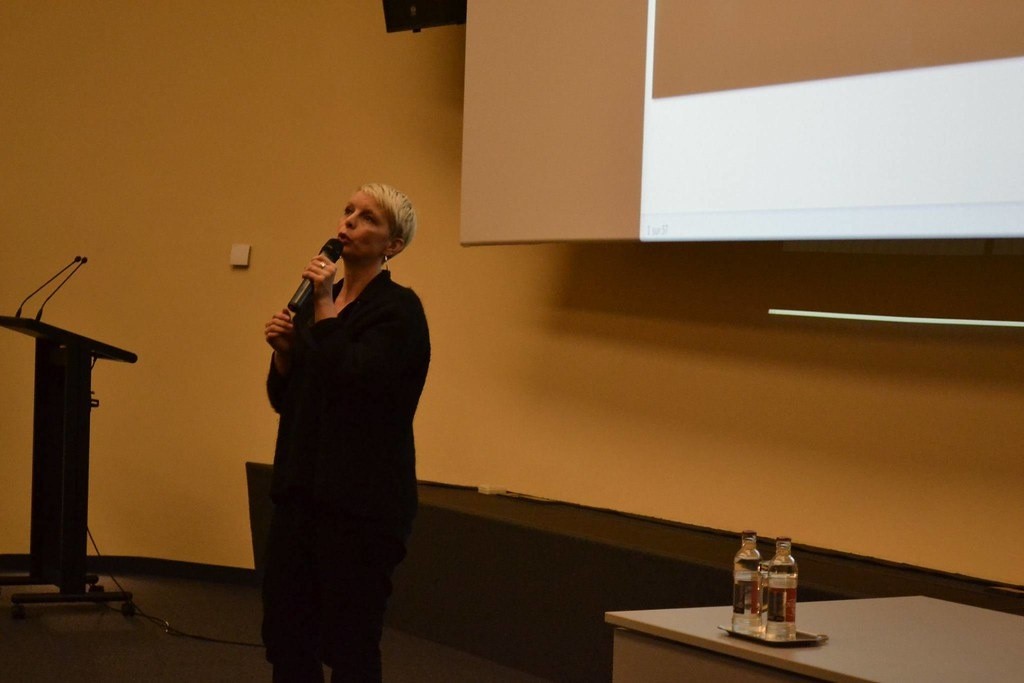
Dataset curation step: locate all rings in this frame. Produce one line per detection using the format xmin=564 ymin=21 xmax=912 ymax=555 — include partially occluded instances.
xmin=320 ymin=261 xmax=326 ymax=269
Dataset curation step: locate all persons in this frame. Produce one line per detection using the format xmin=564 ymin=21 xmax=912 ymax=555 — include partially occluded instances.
xmin=258 ymin=185 xmax=432 ymax=683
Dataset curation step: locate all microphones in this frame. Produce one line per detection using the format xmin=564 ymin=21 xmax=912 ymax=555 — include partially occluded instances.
xmin=288 ymin=238 xmax=343 ymax=314
xmin=36 ymin=257 xmax=88 ymax=321
xmin=15 ymin=256 xmax=81 ymax=318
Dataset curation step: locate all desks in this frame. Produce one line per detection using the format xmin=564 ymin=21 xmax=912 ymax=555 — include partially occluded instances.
xmin=604 ymin=594 xmax=1024 ymax=682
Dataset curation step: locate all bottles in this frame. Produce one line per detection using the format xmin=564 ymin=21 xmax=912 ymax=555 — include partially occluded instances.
xmin=766 ymin=535 xmax=798 ymax=640
xmin=732 ymin=529 xmax=765 ymax=637
xmin=754 ymin=557 xmax=774 ymax=636
